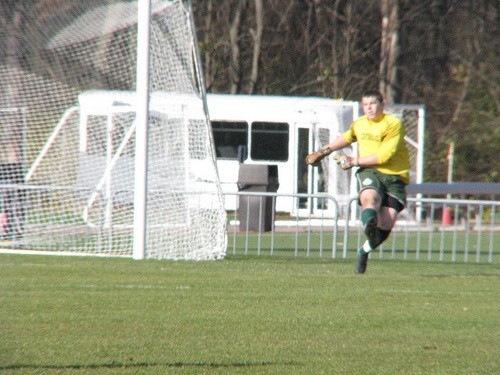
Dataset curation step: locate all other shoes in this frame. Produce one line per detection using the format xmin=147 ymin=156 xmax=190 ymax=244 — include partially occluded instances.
xmin=362 ymin=216 xmax=377 ymax=241
xmin=355 ymin=247 xmax=367 ymax=274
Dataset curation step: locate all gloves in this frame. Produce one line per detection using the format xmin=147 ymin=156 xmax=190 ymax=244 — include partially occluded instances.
xmin=333 ymin=154 xmax=359 ymax=170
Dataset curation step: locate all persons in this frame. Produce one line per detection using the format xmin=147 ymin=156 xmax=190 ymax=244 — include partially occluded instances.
xmin=306 ymin=90 xmax=410 ymax=273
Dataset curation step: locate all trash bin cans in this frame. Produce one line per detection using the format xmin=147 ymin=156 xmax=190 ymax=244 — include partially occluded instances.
xmin=0 ymin=161 xmax=27 ymax=241
xmin=235 ymin=163 xmax=280 ymax=233
